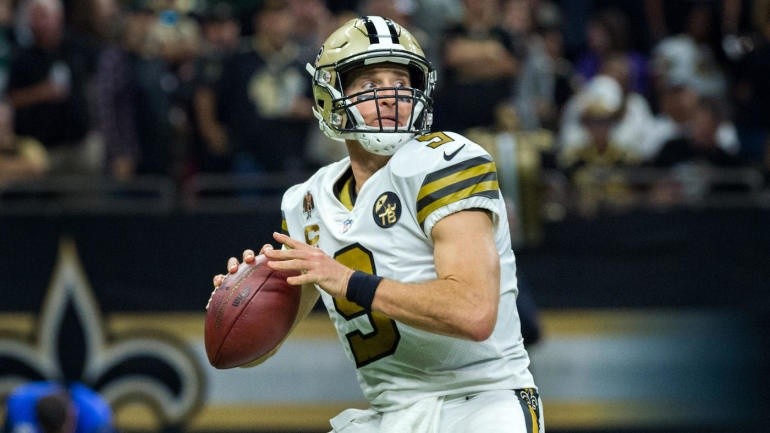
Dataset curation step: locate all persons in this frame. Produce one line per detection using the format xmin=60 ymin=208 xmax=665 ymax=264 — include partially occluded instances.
xmin=213 ymin=15 xmax=545 ymax=433
xmin=0 ymin=0 xmax=770 ymax=213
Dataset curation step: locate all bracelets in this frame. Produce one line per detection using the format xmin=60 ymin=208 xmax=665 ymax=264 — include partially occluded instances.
xmin=345 ymin=269 xmax=384 ymax=312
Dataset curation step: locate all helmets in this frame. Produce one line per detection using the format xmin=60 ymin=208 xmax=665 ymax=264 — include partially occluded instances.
xmin=576 ymin=75 xmax=622 ymax=123
xmin=652 ymin=36 xmax=699 ymax=90
xmin=312 ymin=15 xmax=437 ymax=155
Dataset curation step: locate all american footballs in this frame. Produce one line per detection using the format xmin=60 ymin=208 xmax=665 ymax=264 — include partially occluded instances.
xmin=204 ymin=249 xmax=301 ymax=370
